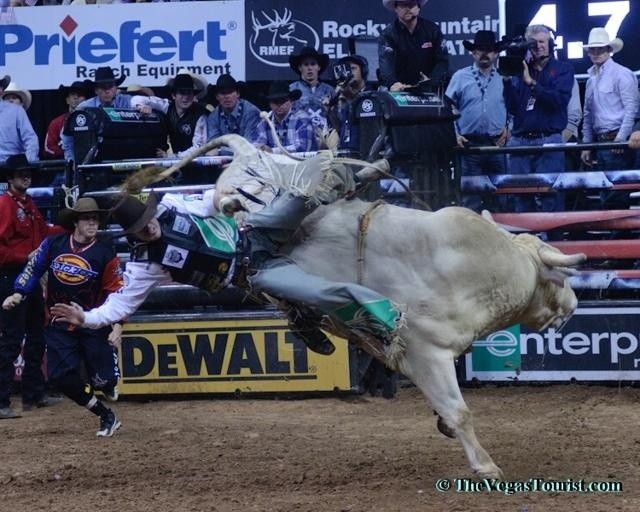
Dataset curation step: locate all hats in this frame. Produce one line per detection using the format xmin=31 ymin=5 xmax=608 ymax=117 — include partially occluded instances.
xmin=108 ymin=191 xmax=158 ymax=238
xmin=463 ymin=30 xmax=511 ymax=53
xmin=58 ymin=67 xmax=126 ymax=97
xmin=260 ymin=81 xmax=301 ymax=102
xmin=207 ymin=73 xmax=247 ymax=94
xmin=168 ymin=73 xmax=204 ymax=96
xmin=0 ymin=75 xmax=32 ymax=111
xmin=1 ymin=154 xmax=41 ymax=183
xmin=59 ymin=198 xmax=110 ymax=230
xmin=290 ymin=48 xmax=329 ymax=74
xmin=383 ymin=0 xmax=427 ymax=12
xmin=582 ymin=28 xmax=624 ymax=54
xmin=343 ymin=54 xmax=369 ymax=80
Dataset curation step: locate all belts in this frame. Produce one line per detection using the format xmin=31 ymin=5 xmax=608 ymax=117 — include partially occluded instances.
xmin=522 ymin=130 xmax=556 ymax=140
xmin=597 ymin=129 xmax=619 ymax=141
xmin=212 ymin=262 xmax=228 ymax=289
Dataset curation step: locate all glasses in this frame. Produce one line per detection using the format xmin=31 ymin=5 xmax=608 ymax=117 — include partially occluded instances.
xmin=396 ymin=1 xmax=417 ymax=8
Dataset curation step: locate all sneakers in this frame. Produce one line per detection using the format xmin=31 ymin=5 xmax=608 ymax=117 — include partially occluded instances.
xmin=0 ymin=408 xmax=22 ymax=419
xmin=24 ymin=397 xmax=63 ymax=409
xmin=96 ymin=409 xmax=122 ymax=438
xmin=104 ymin=385 xmax=119 ymax=402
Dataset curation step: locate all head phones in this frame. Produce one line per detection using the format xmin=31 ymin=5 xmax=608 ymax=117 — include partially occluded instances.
xmin=352 ymin=53 xmax=369 ymax=81
xmin=542 ymin=24 xmax=557 ymax=57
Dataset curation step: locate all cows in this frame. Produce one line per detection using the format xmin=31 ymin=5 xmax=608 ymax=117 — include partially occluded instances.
xmin=111 ymin=132 xmax=589 ymax=482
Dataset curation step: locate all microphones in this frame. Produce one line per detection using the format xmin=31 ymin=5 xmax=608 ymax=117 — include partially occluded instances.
xmin=540 ymin=54 xmax=550 ymax=61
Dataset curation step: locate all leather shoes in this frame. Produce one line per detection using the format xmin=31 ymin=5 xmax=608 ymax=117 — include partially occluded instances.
xmin=355 ymin=159 xmax=390 ymax=186
xmin=289 ymin=320 xmax=335 ymax=354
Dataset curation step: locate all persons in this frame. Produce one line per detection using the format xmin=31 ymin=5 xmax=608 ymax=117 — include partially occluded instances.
xmin=0 ymin=1 xmax=639 ymax=239
xmin=0 ymin=156 xmax=65 ymax=419
xmin=49 ymin=158 xmax=391 ymax=355
xmin=3 ymin=196 xmax=125 ymax=437
